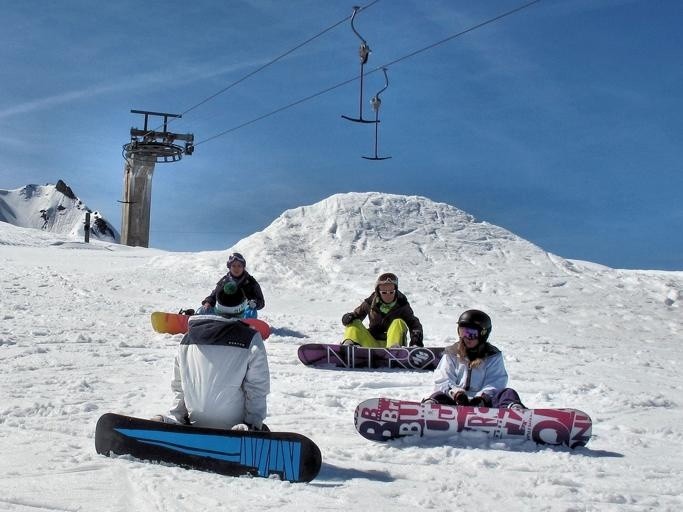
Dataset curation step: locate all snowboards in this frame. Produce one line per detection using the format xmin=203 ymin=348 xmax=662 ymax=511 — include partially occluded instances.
xmin=298 ymin=344 xmax=446 ymax=373
xmin=151 ymin=310 xmax=272 ymax=340
xmin=94 ymin=413 xmax=321 ymax=482
xmin=353 ymin=399 xmax=592 ymax=450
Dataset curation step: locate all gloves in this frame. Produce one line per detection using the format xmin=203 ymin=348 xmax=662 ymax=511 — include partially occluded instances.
xmin=342 ymin=313 xmax=355 ymax=325
xmin=249 ymin=299 xmax=257 ymax=309
xmin=454 ymin=392 xmax=469 ymax=406
xmin=409 ymin=336 xmax=423 ymax=347
xmin=469 ymin=396 xmax=488 ymax=408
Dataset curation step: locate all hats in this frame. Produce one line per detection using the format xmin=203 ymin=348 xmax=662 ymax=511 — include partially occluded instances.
xmin=213 ymin=282 xmax=248 ymax=315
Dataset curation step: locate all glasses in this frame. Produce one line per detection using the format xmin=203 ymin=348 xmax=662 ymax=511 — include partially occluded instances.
xmin=458 ymin=326 xmax=488 ymax=339
xmin=229 ymin=253 xmax=245 ymax=263
xmin=379 ymin=291 xmax=394 ymax=295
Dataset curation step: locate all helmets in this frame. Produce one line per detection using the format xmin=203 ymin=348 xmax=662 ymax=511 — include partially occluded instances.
xmin=227 ymin=253 xmax=246 ymax=269
xmin=375 ymin=273 xmax=398 ymax=290
xmin=457 ymin=310 xmax=491 ymax=345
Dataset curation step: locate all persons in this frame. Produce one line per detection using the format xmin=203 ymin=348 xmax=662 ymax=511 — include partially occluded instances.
xmin=198 ymin=250 xmax=266 ymax=319
xmin=419 ymin=307 xmax=524 ymax=409
xmin=151 ymin=281 xmax=271 ymax=435
xmin=339 ymin=273 xmax=424 ymax=349
